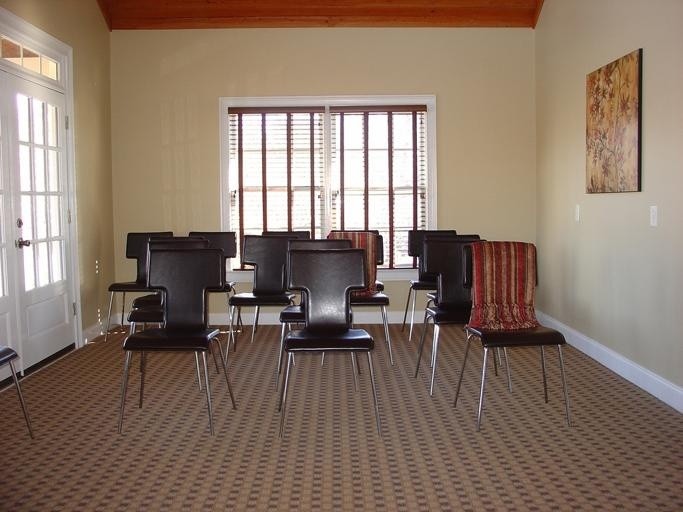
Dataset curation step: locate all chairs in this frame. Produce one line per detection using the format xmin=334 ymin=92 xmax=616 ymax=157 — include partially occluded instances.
xmin=450 ymin=240 xmax=573 ymax=433
xmin=0 ymin=345 xmax=35 ymax=439
xmin=102 ymin=230 xmax=574 ymax=391
xmin=276 ymin=246 xmax=387 ymax=437
xmin=115 ymin=246 xmax=237 ymax=440
